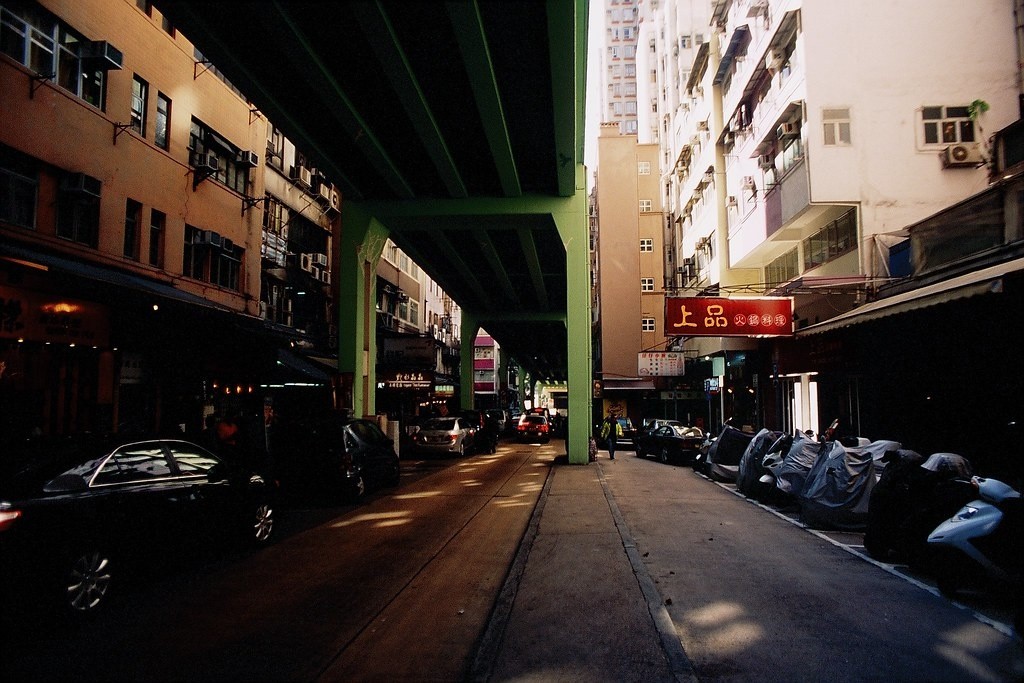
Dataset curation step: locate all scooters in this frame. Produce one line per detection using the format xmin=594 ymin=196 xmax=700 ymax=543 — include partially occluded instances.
xmin=692 ymin=417 xmax=979 ymax=574
xmin=927 ymin=474 xmax=1024 ymax=594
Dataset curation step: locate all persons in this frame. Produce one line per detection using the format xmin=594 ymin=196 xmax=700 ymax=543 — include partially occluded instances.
xmin=600 ymin=411 xmax=623 ymax=460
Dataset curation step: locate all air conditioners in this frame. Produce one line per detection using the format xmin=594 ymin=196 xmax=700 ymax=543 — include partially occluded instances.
xmin=329 ymin=190 xmax=338 ymax=207
xmin=294 ymin=166 xmax=311 ymax=188
xmin=316 ymin=183 xmax=330 ymax=201
xmin=675 ymin=85 xmax=713 ymax=217
xmin=194 ymin=230 xmax=220 ymax=248
xmin=64 ymin=172 xmax=103 ymax=199
xmin=198 ymin=154 xmax=218 ymax=172
xmin=740 ymin=176 xmax=754 ymax=190
xmin=724 ymin=134 xmax=733 ymax=144
xmin=945 ymin=144 xmax=983 ymax=166
xmin=677 ymin=266 xmax=684 ymax=273
xmin=82 ymin=40 xmax=123 ymax=70
xmin=220 ymin=237 xmax=233 ymax=252
xmin=730 ymin=118 xmax=739 ymax=132
xmin=777 ymin=122 xmax=798 ymax=140
xmin=296 ymin=253 xmax=311 ymax=272
xmin=241 ymin=150 xmax=258 ymax=168
xmin=757 ymin=155 xmax=775 ymax=168
xmin=683 ymin=258 xmax=691 ymax=265
xmin=314 ymin=253 xmax=327 ymax=266
xmin=766 ymin=50 xmax=784 ymax=69
xmin=726 ymin=196 xmax=735 ymax=207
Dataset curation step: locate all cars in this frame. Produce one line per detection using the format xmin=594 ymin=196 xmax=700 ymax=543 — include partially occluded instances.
xmin=0 ymin=431 xmax=280 ymax=628
xmin=597 ymin=416 xmax=710 ymax=465
xmin=472 ymin=407 xmax=554 ymax=455
xmin=267 ymin=419 xmax=402 ymax=505
xmin=411 ymin=415 xmax=478 ymax=458
